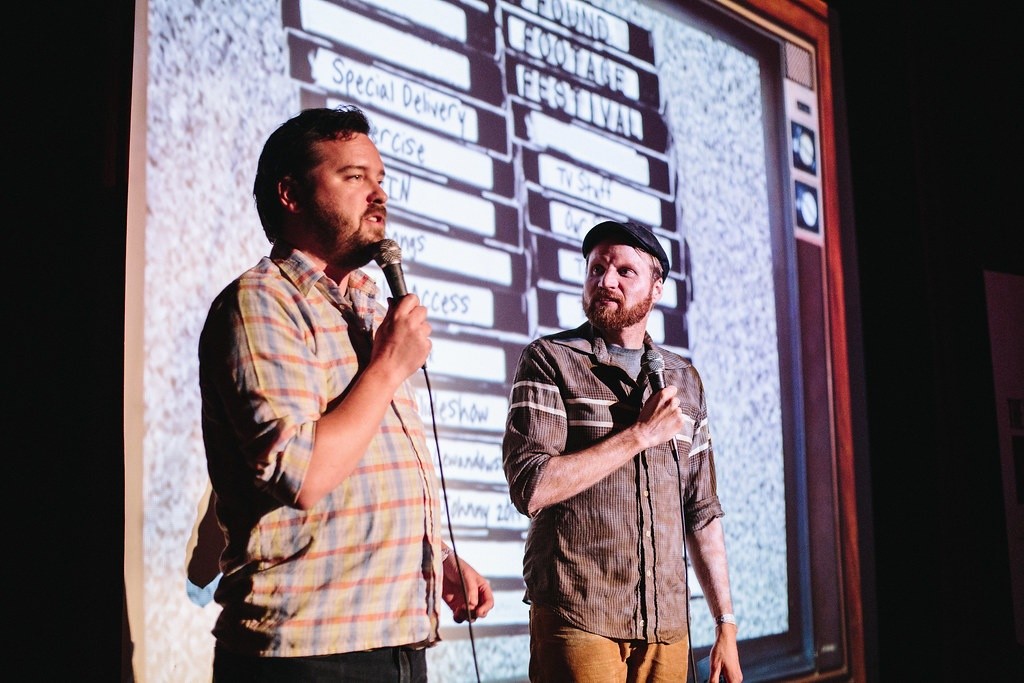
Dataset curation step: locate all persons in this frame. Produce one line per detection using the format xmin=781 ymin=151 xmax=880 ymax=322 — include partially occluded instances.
xmin=503 ymin=222 xmax=743 ymax=683
xmin=198 ymin=106 xmax=494 ymax=683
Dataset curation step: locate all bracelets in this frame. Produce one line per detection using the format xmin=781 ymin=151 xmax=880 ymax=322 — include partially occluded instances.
xmin=714 ymin=615 xmax=737 ymax=627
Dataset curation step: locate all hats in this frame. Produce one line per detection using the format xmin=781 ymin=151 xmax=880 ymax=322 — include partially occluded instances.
xmin=582 ymin=221 xmax=670 ymax=284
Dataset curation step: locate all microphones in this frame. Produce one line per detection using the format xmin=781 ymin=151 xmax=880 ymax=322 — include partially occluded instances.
xmin=642 ymin=350 xmax=679 ymax=462
xmin=375 ymin=239 xmax=428 ymax=370
